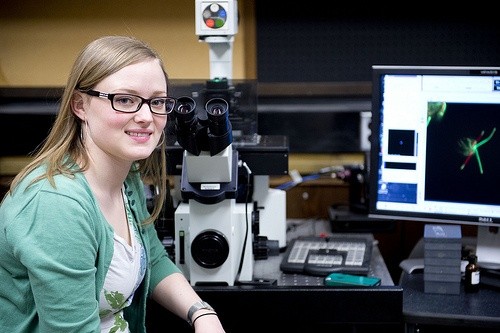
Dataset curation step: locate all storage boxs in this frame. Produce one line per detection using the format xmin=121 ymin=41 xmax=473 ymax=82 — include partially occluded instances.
xmin=424 ymin=224 xmax=461 ymax=295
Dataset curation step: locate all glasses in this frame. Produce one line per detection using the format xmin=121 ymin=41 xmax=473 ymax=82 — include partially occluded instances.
xmin=81 ymin=87 xmax=177 ymax=115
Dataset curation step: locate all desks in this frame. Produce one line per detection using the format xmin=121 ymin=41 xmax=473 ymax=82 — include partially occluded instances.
xmin=145 ymin=234 xmax=404 ymax=333
xmin=399 ymin=239 xmax=500 ymax=321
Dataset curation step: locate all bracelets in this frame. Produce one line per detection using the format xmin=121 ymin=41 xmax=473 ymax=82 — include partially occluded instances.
xmin=187 ymin=301 xmax=214 ymax=326
xmin=192 ymin=312 xmax=217 ymax=327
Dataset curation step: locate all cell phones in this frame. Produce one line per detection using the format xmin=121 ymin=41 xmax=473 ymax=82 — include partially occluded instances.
xmin=324 ymin=272 xmax=382 ymax=289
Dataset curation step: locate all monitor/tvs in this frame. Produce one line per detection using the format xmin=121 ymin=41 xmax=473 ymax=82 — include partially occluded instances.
xmin=367 ymin=66 xmax=500 ymax=276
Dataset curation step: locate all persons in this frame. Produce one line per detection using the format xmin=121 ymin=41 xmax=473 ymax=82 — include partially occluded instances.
xmin=0 ymin=35 xmax=225 ymax=333
